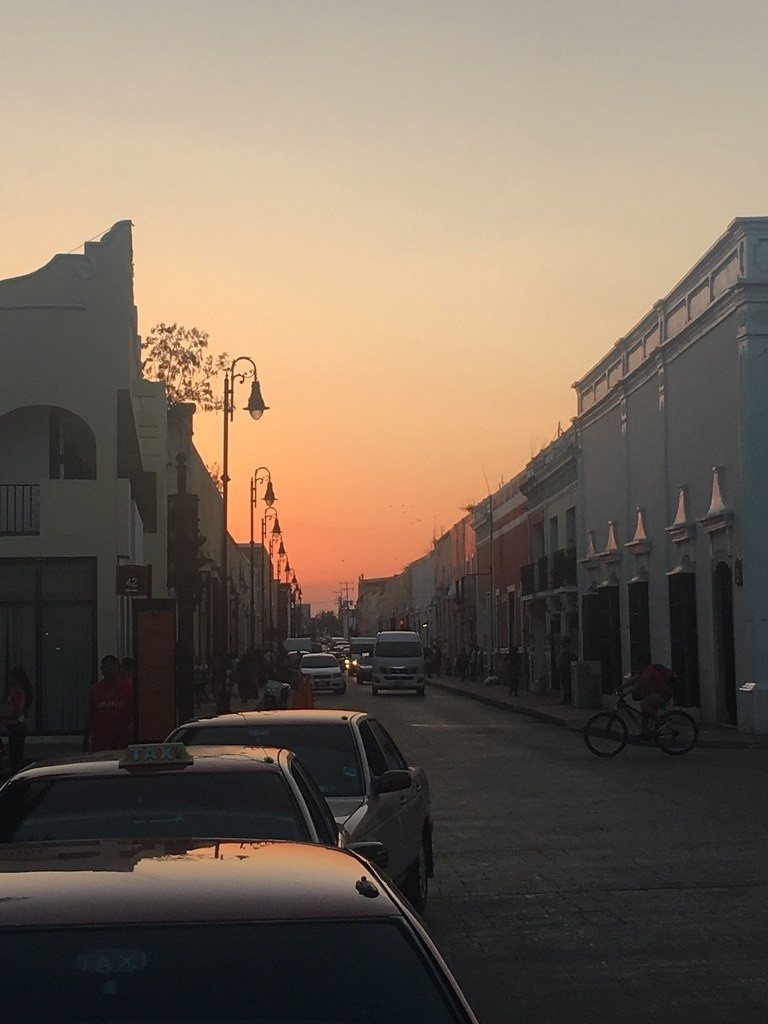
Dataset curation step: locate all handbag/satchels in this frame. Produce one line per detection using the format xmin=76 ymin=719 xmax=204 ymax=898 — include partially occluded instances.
xmin=81 ymin=735 xmax=101 ymax=753
xmin=654 ymin=663 xmax=676 ymax=686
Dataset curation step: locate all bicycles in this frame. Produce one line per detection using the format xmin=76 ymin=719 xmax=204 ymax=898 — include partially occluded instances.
xmin=582 ymin=676 xmax=702 ymax=757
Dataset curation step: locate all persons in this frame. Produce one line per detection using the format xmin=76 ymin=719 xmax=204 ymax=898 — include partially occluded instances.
xmin=190 ymin=654 xmax=261 ymax=714
xmin=83 ymin=650 xmax=134 ymax=751
xmin=558 ymin=636 xmax=579 ymax=705
xmin=6 ymin=665 xmax=34 ymax=775
xmin=504 ymin=644 xmax=523 ymax=697
xmin=427 ymin=645 xmax=443 ymax=679
xmin=456 ymin=647 xmax=467 ymax=682
xmin=615 ymin=656 xmax=677 ymax=738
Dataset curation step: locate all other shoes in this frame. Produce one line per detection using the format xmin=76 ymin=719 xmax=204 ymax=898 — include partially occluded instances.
xmin=642 ymin=736 xmax=656 ymax=744
xmin=508 ymin=688 xmax=513 ymax=696
xmin=515 ymin=693 xmax=519 ymax=696
xmin=427 ymin=676 xmax=432 ymax=678
xmin=437 ymin=675 xmax=440 ymax=677
xmin=654 ymin=720 xmax=668 ymax=732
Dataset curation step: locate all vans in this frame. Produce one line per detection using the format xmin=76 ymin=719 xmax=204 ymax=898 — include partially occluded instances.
xmin=346 ymin=637 xmax=380 ymax=676
xmin=372 ymin=632 xmax=433 ymax=694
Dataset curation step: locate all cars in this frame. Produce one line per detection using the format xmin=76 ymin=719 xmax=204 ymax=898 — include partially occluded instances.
xmin=357 ymin=653 xmax=376 ymax=685
xmin=1 ymin=746 xmax=389 ymax=877
xmin=152 ymin=708 xmax=437 ymax=910
xmin=1 ymin=836 xmax=475 ymax=1023
xmin=311 ymin=635 xmax=349 ymax=670
xmin=299 ymin=653 xmax=348 ymax=693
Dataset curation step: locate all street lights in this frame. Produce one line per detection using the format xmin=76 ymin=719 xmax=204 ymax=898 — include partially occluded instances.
xmin=267 ymin=534 xmax=304 ymax=640
xmin=217 ymin=357 xmax=272 ymax=712
xmin=249 ymin=466 xmax=280 ymax=649
xmin=261 ymin=506 xmax=284 ymax=642
